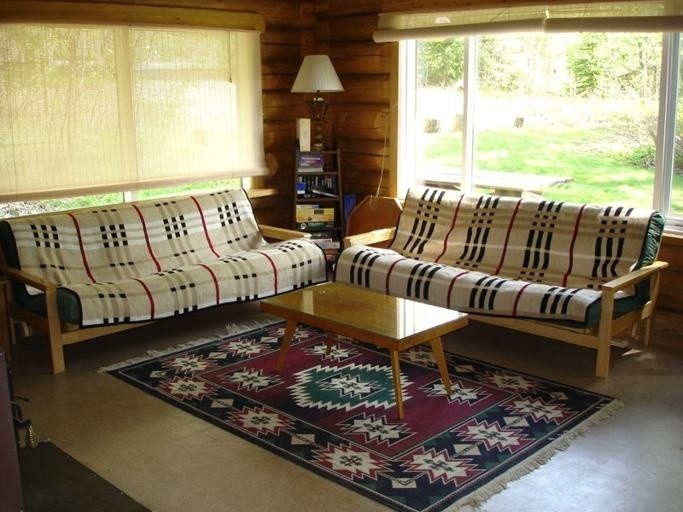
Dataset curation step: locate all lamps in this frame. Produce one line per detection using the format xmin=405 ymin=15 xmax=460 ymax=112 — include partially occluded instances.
xmin=289 ymin=53 xmax=346 ymax=150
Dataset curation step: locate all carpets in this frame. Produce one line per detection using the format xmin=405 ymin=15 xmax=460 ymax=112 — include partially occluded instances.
xmin=20 ymin=435 xmax=151 ymax=511
xmin=94 ymin=312 xmax=624 ymax=511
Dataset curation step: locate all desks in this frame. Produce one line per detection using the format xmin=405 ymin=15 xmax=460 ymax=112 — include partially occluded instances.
xmin=255 ymin=278 xmax=472 ymax=426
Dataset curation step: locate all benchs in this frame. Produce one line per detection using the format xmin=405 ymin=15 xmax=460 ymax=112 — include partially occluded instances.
xmin=332 ymin=180 xmax=671 ymax=381
xmin=1 ymin=186 xmax=329 ymax=377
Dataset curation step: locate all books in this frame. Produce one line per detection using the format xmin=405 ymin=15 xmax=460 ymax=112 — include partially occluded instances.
xmin=297 ymin=155 xmax=338 ymax=198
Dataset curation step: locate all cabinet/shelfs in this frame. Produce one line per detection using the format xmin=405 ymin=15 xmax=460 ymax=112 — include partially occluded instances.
xmin=288 ymin=150 xmax=346 ymax=270
